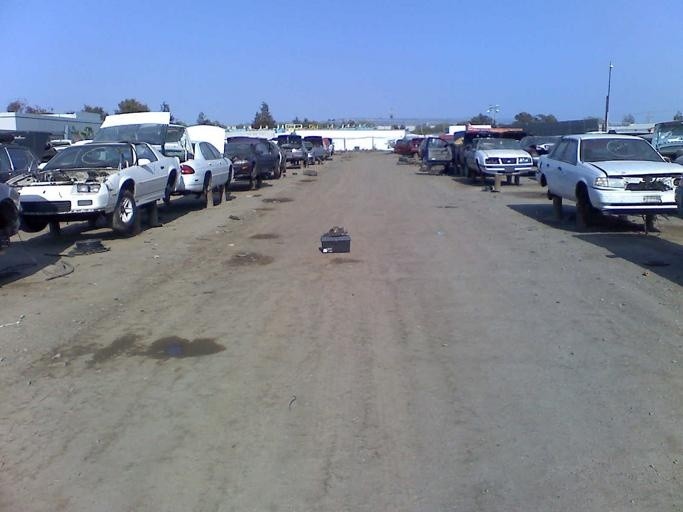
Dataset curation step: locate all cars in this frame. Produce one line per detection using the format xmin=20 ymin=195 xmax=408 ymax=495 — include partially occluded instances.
xmin=133 ymin=124 xmax=333 ymax=202
xmin=0 ymin=181 xmax=21 ymax=246
xmin=0 ymin=130 xmax=50 ymax=182
xmin=533 ymin=129 xmax=683 ymax=218
xmin=48 ymin=136 xmax=89 ymax=151
xmin=392 ymin=120 xmax=683 ymax=178
xmin=6 ymin=111 xmax=181 ymax=234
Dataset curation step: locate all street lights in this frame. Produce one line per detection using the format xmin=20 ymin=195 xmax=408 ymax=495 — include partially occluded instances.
xmin=486 ymin=102 xmax=500 ymax=124
xmin=602 ymin=59 xmax=613 ymax=131
